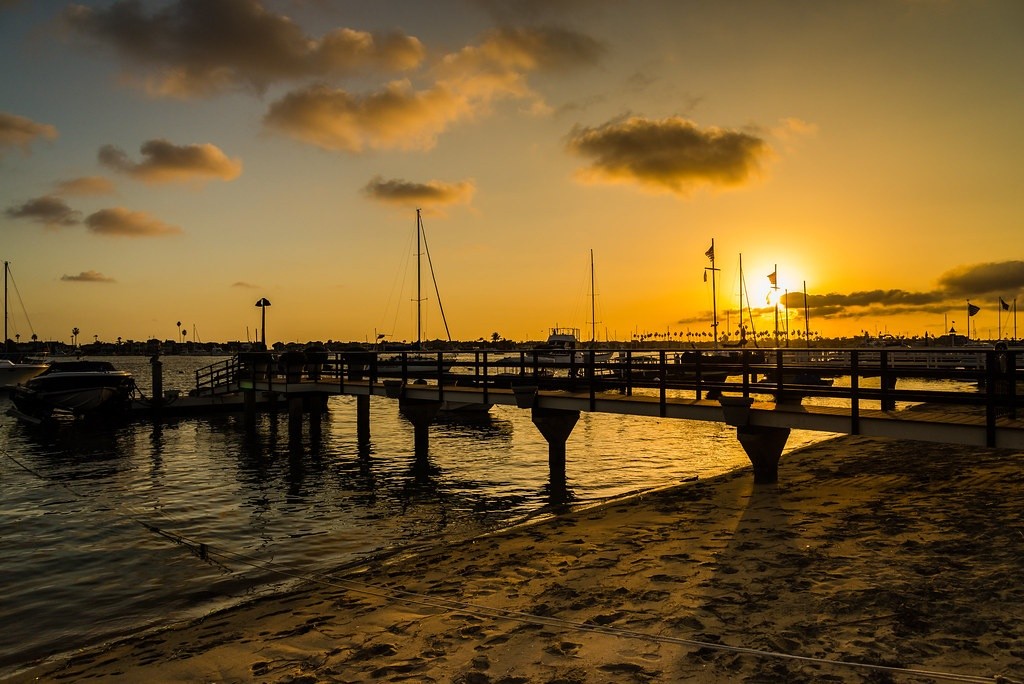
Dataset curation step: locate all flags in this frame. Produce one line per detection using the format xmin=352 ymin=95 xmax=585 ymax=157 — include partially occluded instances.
xmin=969 ymin=304 xmax=980 ymax=316
xmin=706 ymin=247 xmax=714 ymax=261
xmin=767 ymin=272 xmax=776 ymax=284
xmin=1001 ymin=299 xmax=1009 ymax=310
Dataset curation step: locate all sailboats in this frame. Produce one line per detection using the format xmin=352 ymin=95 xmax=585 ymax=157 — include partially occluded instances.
xmin=362 ymin=207 xmax=457 ymax=376
xmin=0 ymin=260 xmax=50 ymax=393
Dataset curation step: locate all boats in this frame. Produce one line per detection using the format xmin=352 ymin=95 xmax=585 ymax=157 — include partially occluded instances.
xmin=398 ymin=374 xmax=502 ymax=412
xmin=497 ymin=238 xmax=1024 ymax=385
xmin=4 ymin=360 xmax=134 ymax=431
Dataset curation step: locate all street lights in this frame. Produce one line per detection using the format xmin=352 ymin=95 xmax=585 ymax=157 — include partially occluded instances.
xmin=255 ymin=297 xmax=272 ymax=344
